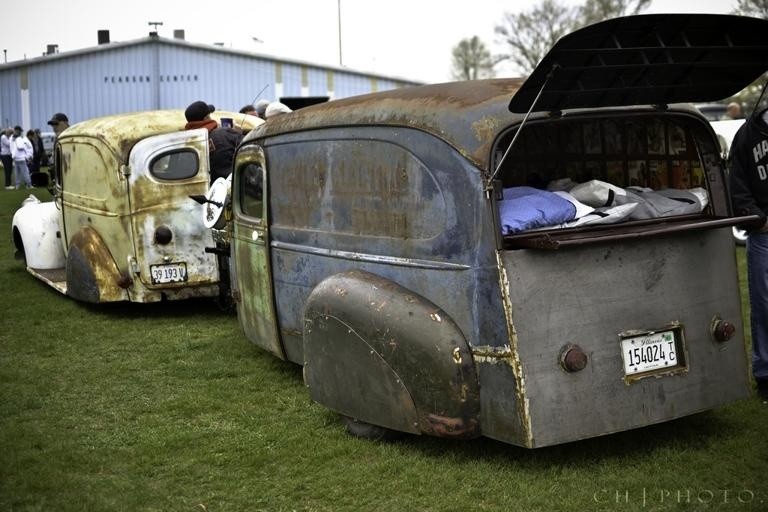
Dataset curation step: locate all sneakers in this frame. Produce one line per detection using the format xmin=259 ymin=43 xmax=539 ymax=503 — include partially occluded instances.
xmin=26 ymin=185 xmax=34 ymax=189
xmin=5 ymin=185 xmax=16 ymax=190
xmin=756 ymin=376 xmax=768 ymax=405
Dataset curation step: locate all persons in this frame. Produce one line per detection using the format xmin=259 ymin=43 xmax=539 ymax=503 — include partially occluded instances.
xmin=47 ymin=112 xmax=70 ymax=186
xmin=720 ymin=102 xmax=740 ymax=120
xmin=0 ymin=125 xmax=48 ymax=190
xmin=171 ymin=101 xmax=243 ymax=187
xmin=726 ymin=107 xmax=767 ymax=408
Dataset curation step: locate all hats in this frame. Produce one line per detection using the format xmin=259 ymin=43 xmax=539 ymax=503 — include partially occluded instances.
xmin=47 ymin=113 xmax=68 ymax=125
xmin=185 ymin=101 xmax=215 ymax=123
xmin=15 ymin=126 xmax=23 ymax=131
